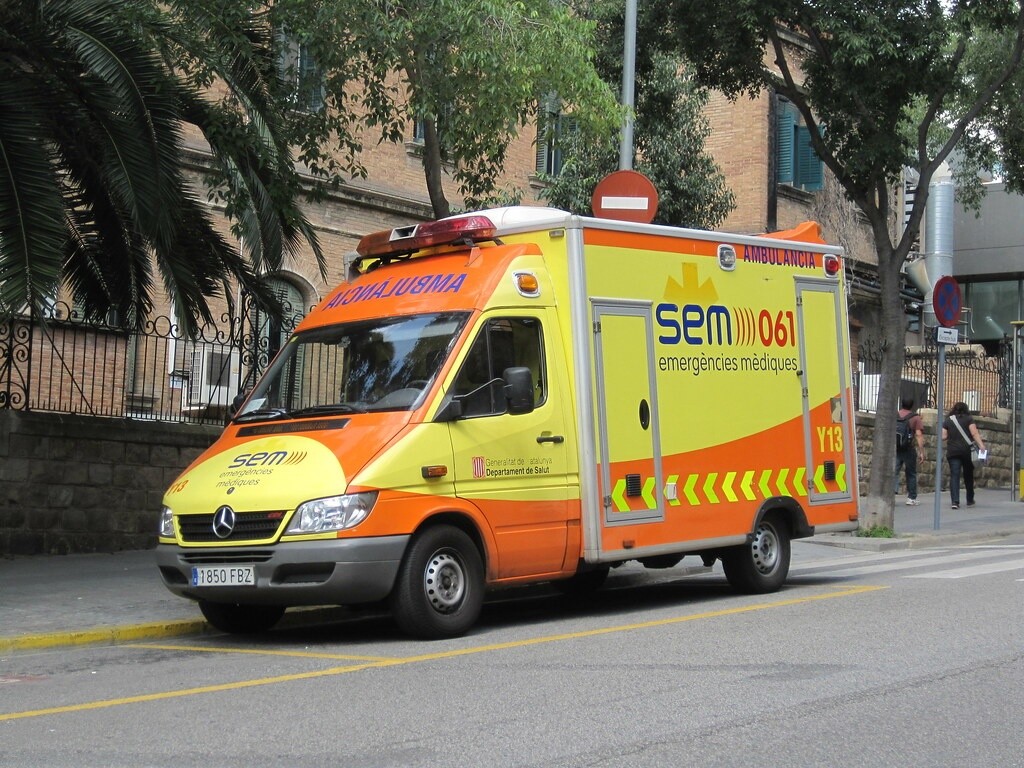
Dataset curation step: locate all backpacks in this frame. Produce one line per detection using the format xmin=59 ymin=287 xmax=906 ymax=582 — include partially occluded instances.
xmin=896 ymin=412 xmax=918 ymax=451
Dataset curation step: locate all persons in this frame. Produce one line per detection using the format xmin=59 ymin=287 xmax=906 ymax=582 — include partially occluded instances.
xmin=895 ymin=397 xmax=924 ymax=505
xmin=941 ymin=402 xmax=985 ymax=509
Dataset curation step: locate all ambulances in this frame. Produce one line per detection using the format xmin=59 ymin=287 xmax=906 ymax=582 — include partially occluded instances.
xmin=153 ymin=206 xmax=861 ymax=639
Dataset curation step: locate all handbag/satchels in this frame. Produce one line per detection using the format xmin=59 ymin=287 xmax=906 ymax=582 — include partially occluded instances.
xmin=971 ymin=441 xmax=982 ymax=468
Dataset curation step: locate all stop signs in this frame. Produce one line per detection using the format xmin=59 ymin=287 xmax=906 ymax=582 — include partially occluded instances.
xmin=591 ymin=172 xmax=658 ymax=225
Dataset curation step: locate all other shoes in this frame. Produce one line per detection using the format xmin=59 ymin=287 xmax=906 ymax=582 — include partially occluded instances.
xmin=968 ymin=503 xmax=975 ymax=507
xmin=952 ymin=502 xmax=959 ymax=509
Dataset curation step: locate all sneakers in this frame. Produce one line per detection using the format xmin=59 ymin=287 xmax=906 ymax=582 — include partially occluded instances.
xmin=906 ymin=497 xmax=920 ymax=506
